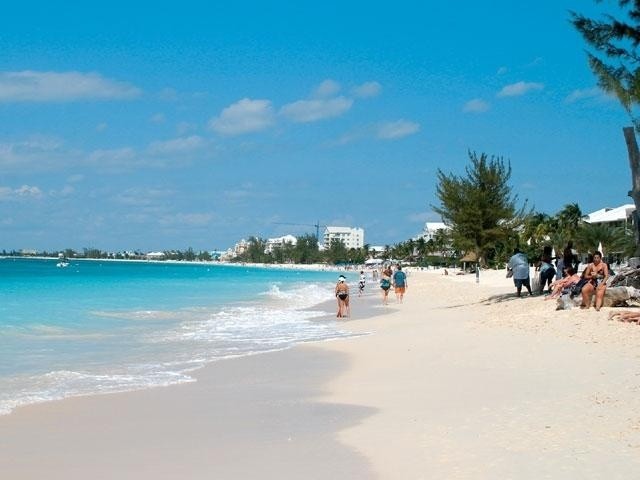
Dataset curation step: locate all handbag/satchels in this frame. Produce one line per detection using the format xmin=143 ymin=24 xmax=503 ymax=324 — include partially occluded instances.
xmin=531 ymin=268 xmax=542 ymax=295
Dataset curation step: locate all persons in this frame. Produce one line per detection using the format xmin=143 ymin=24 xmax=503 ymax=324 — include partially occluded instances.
xmin=335 ymin=277 xmax=348 ymax=318
xmin=506 ymin=237 xmax=611 ymax=310
xmin=336 ymin=275 xmax=351 ymax=318
xmin=358 ymin=260 xmax=409 ymax=303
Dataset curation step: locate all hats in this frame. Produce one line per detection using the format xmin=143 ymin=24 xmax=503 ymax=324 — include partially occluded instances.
xmin=338 ymin=274 xmax=347 ymax=282
